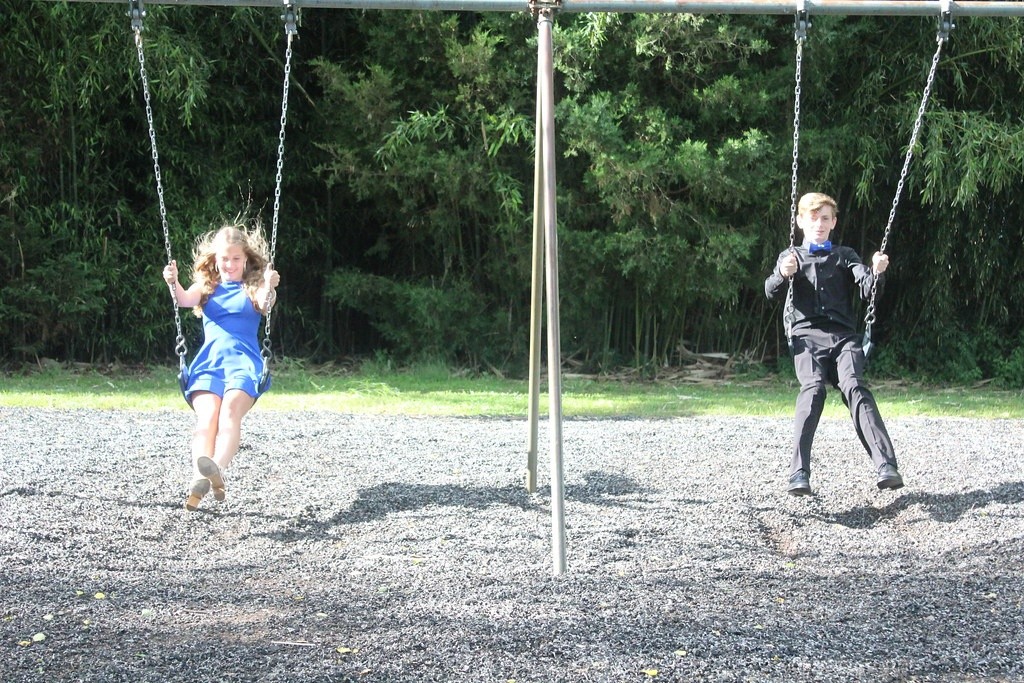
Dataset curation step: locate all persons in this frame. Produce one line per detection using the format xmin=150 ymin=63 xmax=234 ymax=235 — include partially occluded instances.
xmin=764 ymin=191 xmax=904 ymax=495
xmin=162 ymin=221 xmax=281 ymax=511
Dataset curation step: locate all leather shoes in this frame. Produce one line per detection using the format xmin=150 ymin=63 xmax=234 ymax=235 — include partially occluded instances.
xmin=787 ymin=470 xmax=811 ymax=497
xmin=876 ymin=464 xmax=904 ymax=490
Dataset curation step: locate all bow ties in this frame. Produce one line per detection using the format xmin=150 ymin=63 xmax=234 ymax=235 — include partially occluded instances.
xmin=808 ymin=240 xmax=832 ymax=254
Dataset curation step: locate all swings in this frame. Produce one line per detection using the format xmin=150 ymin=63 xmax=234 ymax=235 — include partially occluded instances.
xmin=785 ymin=17 xmax=954 ymax=382
xmin=121 ymin=1 xmax=302 ymax=417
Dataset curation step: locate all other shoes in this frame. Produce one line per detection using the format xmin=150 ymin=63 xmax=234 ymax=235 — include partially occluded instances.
xmin=186 ymin=478 xmax=210 ymax=510
xmin=197 ymin=456 xmax=225 ymax=501
